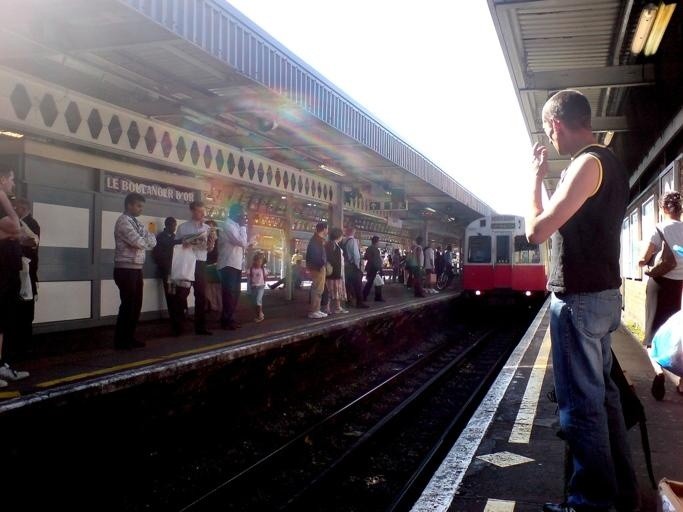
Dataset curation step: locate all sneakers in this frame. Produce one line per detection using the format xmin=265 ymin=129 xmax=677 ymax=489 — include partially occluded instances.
xmin=0 ymin=363 xmax=30 ymax=380
xmin=254 ymin=313 xmax=265 ymax=323
xmin=423 ymin=288 xmax=439 ymax=294
xmin=308 ymin=307 xmax=349 ymax=318
xmin=542 ymin=501 xmax=587 ymax=512
xmin=0 ymin=380 xmax=8 ymax=388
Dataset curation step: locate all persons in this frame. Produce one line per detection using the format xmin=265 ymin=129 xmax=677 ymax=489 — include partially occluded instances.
xmin=1 ymin=163 xmax=40 ymax=388
xmin=523 ymin=85 xmax=645 ymax=511
xmin=111 ymin=193 xmax=269 ymax=348
xmin=268 ymin=221 xmax=455 ymax=318
xmin=637 ymin=189 xmax=683 ymax=402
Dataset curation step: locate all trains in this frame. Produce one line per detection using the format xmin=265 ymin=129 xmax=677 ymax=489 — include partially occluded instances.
xmin=206 ymin=220 xmax=401 ymax=280
xmin=462 ymin=214 xmax=553 ymax=310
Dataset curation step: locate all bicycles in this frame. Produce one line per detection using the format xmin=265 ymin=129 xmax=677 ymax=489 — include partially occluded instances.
xmin=435 ymin=269 xmax=449 ymax=291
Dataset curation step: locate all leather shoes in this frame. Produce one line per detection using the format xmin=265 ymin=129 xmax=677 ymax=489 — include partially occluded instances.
xmin=196 ymin=321 xmax=240 ymax=335
xmin=357 ymin=303 xmax=371 ymax=308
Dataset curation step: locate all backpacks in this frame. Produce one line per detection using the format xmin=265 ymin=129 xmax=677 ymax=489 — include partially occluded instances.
xmin=339 ymin=238 xmax=353 ymax=262
xmin=406 ymin=246 xmax=421 ymax=268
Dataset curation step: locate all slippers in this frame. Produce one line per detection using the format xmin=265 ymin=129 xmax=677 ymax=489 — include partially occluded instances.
xmin=650 ymin=374 xmax=665 ymax=401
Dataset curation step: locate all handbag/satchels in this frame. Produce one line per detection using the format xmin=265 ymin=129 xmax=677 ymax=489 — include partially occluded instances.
xmin=170 ymin=244 xmax=196 ymax=281
xmin=643 ymin=227 xmax=677 ymax=279
xmin=430 ymin=273 xmax=437 ymax=283
xmin=546 ymin=344 xmax=643 ymax=445
xmin=308 ymin=283 xmax=328 ymax=305
